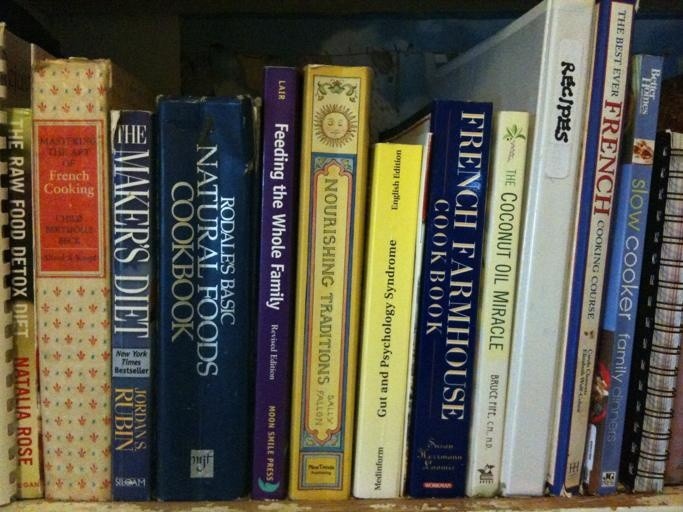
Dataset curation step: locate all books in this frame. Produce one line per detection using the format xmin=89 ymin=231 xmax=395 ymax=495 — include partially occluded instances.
xmin=0 ymin=1 xmax=682 ymax=503
